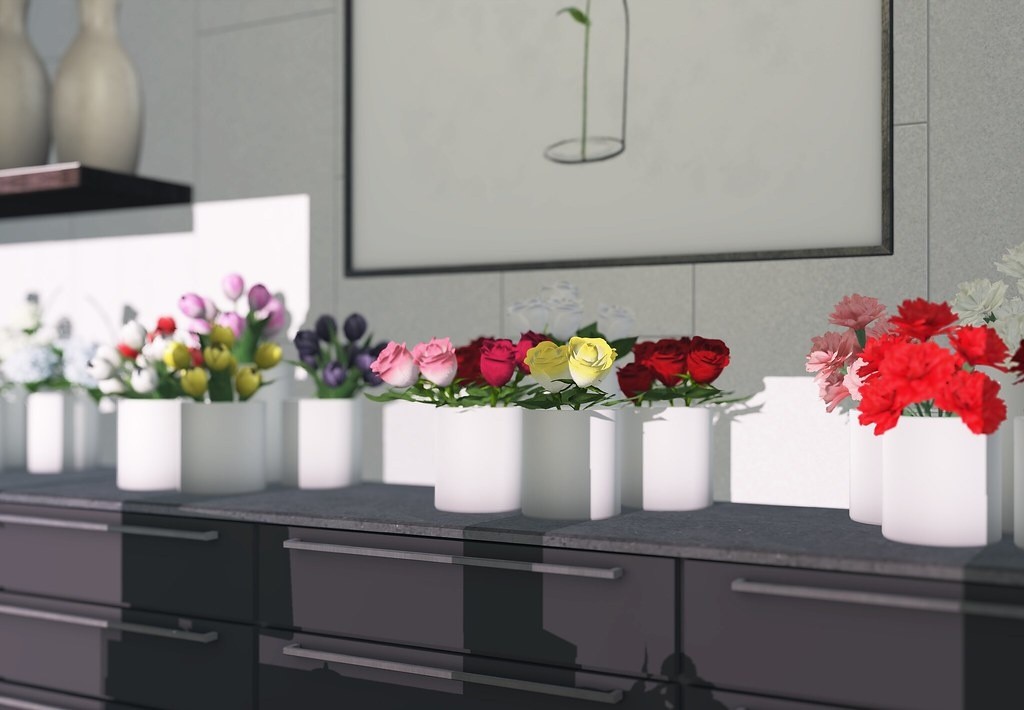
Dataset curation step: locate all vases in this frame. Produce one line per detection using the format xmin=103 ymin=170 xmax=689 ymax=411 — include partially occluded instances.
xmin=0 ymin=377 xmax=1023 ymax=549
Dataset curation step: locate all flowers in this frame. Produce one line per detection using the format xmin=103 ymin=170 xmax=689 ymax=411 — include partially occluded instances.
xmin=2 ymin=243 xmax=1024 ymax=437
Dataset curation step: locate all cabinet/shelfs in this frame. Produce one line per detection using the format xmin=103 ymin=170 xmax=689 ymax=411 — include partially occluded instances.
xmin=0 ymin=502 xmax=1024 ymax=710
